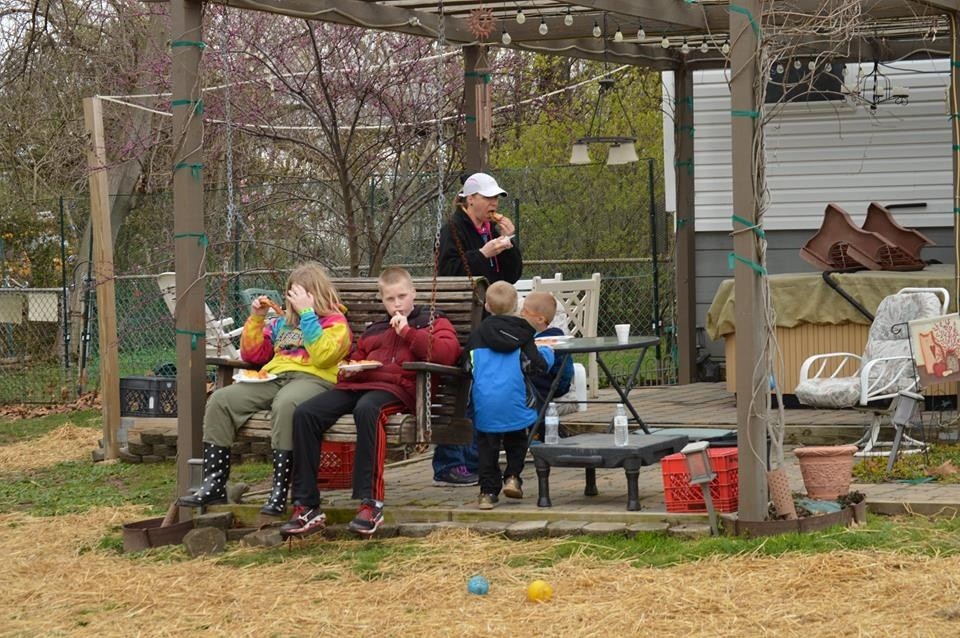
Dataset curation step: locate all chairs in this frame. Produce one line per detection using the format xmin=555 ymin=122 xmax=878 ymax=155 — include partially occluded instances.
xmin=792 ymin=288 xmax=949 ymax=458
xmin=891 ymin=311 xmax=960 ymax=465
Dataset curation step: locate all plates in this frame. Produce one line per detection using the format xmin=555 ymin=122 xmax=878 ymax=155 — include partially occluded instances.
xmin=338 ymin=363 xmax=380 ymax=371
xmin=534 ymin=335 xmax=574 ymax=341
xmin=232 ymin=374 xmax=277 ymax=383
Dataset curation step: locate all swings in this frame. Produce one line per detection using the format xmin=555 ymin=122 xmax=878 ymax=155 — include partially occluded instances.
xmin=207 ymin=56 xmax=493 ymax=445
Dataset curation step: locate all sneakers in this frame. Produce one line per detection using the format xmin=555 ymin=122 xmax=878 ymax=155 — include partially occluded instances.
xmin=348 ymin=498 xmax=386 ymax=533
xmin=432 ymin=464 xmax=480 ymax=486
xmin=280 ymin=504 xmax=327 ymax=533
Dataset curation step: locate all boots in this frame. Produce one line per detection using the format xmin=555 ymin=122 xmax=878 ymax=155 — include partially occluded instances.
xmin=259 ymin=449 xmax=294 ymax=515
xmin=178 ymin=442 xmax=231 ymax=506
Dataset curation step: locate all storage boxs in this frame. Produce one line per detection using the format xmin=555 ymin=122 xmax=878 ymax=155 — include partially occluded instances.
xmin=662 ymin=448 xmax=738 ymax=514
xmin=319 ymin=441 xmax=356 ymax=489
xmin=120 ymin=376 xmax=177 ymax=418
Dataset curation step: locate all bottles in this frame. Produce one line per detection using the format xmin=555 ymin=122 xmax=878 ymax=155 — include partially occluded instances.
xmin=614 ymin=404 xmax=628 ymax=447
xmin=544 ymin=403 xmax=559 ymax=445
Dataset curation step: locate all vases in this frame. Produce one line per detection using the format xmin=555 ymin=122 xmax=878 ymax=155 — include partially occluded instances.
xmin=791 ymin=443 xmax=858 ymax=499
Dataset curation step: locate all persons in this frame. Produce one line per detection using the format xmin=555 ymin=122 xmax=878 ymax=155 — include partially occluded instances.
xmin=437 ymin=172 xmax=574 ymax=509
xmin=274 ymin=267 xmax=461 ymax=533
xmin=174 ymin=265 xmax=357 ymax=516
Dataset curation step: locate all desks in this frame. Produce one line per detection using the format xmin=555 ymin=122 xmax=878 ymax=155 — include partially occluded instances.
xmin=505 ymin=336 xmax=661 ymax=483
xmin=530 ymin=434 xmax=688 ymax=511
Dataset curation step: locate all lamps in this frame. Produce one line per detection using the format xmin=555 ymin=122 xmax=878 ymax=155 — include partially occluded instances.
xmin=569 ymin=14 xmax=640 ymax=166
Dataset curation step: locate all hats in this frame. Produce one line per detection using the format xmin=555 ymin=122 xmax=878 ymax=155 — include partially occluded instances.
xmin=459 ymin=172 xmax=508 ymax=198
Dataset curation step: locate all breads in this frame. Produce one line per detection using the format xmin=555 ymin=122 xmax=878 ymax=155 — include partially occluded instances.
xmin=490 ymin=211 xmax=504 ymax=222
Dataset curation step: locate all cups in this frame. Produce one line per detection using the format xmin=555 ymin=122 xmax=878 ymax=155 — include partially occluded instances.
xmin=614 ymin=324 xmax=630 ymax=344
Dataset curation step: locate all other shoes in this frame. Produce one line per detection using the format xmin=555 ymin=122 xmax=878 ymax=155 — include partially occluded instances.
xmin=478 ymin=492 xmax=501 ymax=509
xmin=503 ymin=477 xmax=524 ymax=499
